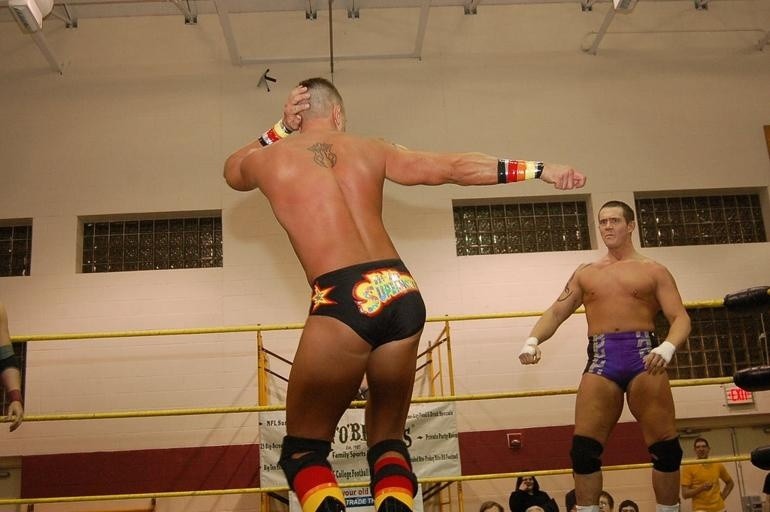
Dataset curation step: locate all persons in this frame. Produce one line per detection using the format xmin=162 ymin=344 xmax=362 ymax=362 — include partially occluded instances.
xmin=681 ymin=437 xmax=734 ymax=511
xmin=520 ymin=197 xmax=691 ymax=512
xmin=0 ymin=303 xmax=24 ymax=431
xmin=224 ymin=77 xmax=587 ymax=512
xmin=480 ymin=474 xmax=640 ymax=512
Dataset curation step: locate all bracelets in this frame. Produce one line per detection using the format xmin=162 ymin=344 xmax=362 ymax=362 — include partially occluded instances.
xmin=258 ymin=118 xmax=293 ymax=148
xmin=497 ymin=158 xmax=544 ymax=183
xmin=7 ymin=389 xmax=23 ymax=404
